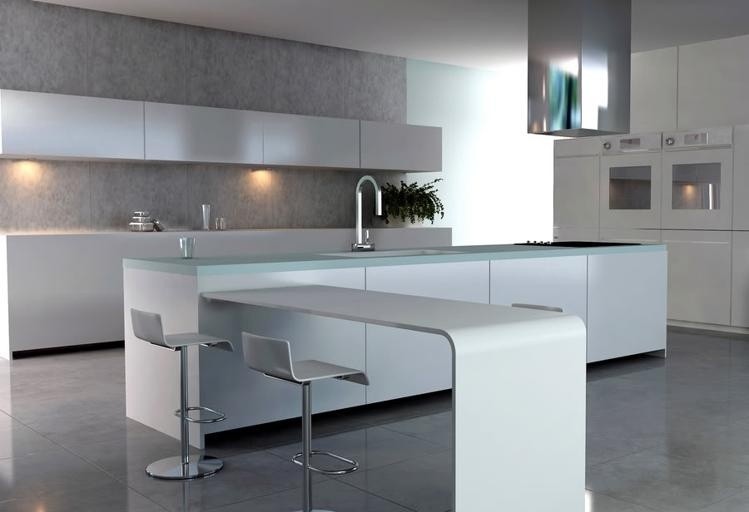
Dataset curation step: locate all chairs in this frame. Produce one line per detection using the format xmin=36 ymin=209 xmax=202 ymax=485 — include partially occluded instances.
xmin=239 ymin=330 xmax=369 ymax=511
xmin=130 ymin=308 xmax=235 ymax=479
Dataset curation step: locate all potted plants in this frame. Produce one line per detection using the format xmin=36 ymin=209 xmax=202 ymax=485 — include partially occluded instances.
xmin=371 ymin=177 xmax=444 ymax=227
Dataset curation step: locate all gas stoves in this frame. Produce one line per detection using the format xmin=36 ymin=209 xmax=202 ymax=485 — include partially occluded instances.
xmin=513 ymin=239 xmax=640 ymax=250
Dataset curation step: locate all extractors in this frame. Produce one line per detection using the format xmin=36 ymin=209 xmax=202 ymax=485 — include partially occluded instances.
xmin=527 ymin=0 xmax=630 ymax=138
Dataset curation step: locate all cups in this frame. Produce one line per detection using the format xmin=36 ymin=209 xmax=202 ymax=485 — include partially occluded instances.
xmin=177 ymin=237 xmax=197 ymax=260
xmin=201 ymin=204 xmax=210 ymax=231
xmin=215 ymin=217 xmax=227 ymax=230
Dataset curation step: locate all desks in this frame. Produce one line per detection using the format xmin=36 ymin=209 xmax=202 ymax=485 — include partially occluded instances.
xmin=199 ymin=284 xmax=587 ymax=512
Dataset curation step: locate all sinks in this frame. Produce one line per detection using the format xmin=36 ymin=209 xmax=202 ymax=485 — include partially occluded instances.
xmin=316 ymin=249 xmax=461 ymax=257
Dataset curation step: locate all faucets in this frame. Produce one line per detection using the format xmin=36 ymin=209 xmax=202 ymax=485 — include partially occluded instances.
xmin=353 ymin=174 xmax=383 ymax=250
